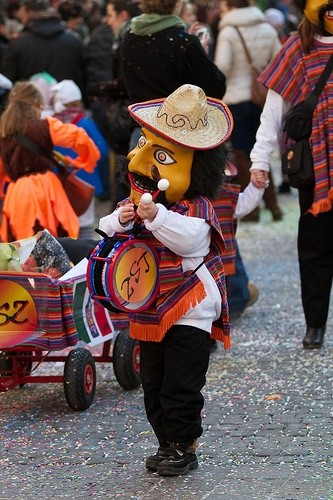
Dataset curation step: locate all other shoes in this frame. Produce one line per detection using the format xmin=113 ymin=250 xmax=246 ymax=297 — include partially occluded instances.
xmin=230 ymin=283 xmax=259 ymax=324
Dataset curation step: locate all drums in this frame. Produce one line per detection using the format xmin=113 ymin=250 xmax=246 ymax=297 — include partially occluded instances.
xmin=85 ymin=233 xmax=161 ymax=314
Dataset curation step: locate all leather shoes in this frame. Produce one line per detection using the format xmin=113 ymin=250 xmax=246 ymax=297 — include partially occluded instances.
xmin=303 ymin=327 xmax=326 ymax=349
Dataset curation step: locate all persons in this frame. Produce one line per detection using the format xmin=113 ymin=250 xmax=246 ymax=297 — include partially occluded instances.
xmin=99 ymin=83 xmax=234 ymax=477
xmin=0 ymin=0 xmax=143 ymax=228
xmin=0 ymin=82 xmax=101 ymax=243
xmin=116 ymin=0 xmax=227 ymax=100
xmin=210 ymin=160 xmax=269 ymax=328
xmin=249 ymin=0 xmax=333 ymax=350
xmin=178 ymin=0 xmax=304 ymax=222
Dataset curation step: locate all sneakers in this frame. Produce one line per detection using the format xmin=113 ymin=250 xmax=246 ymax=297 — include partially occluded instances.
xmin=158 ymin=449 xmax=199 ymax=477
xmin=146 ymin=446 xmax=170 ymax=471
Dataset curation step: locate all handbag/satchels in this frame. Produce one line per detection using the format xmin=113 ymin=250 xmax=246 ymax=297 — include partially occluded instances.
xmin=252 ymin=65 xmax=268 ymax=105
xmin=59 ymin=170 xmax=95 ymax=218
xmin=283 ymin=95 xmax=318 ymax=140
xmin=283 ymin=139 xmax=315 ymax=191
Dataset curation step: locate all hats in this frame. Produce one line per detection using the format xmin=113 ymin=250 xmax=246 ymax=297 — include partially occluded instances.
xmin=51 ymin=80 xmax=82 ymax=112
xmin=30 ymin=73 xmax=57 ymax=108
xmin=128 ymin=83 xmax=234 ymax=150
